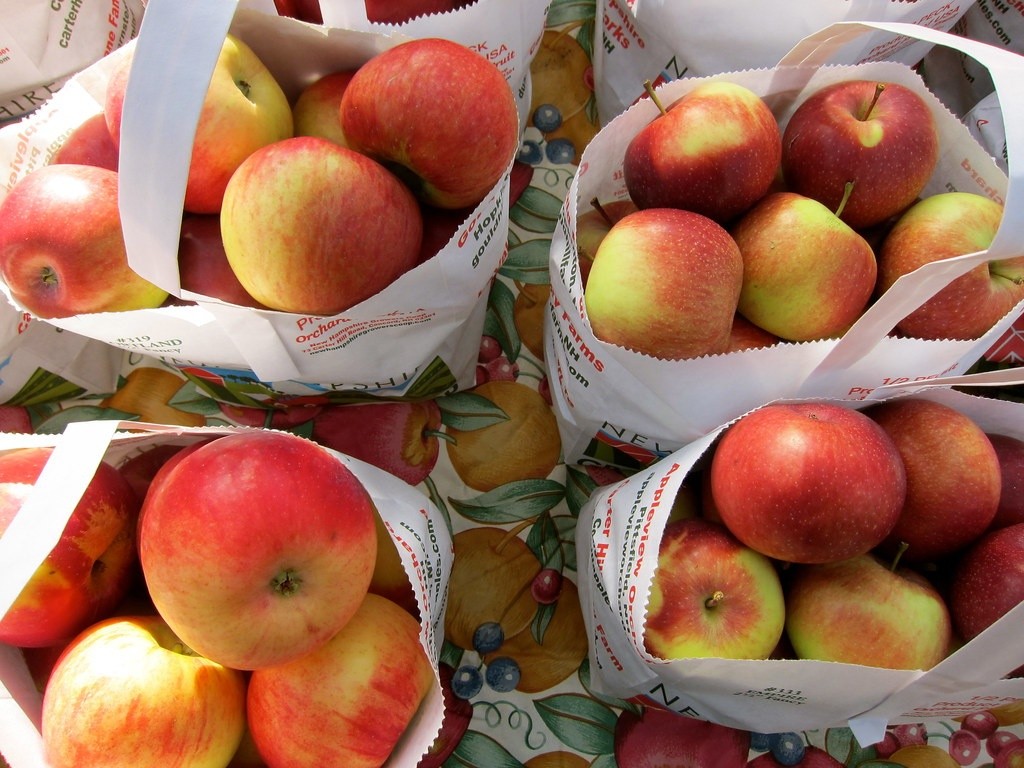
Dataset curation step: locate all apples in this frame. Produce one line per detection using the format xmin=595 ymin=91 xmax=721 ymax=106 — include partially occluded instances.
xmin=643 ymin=398 xmax=1024 ymax=678
xmin=0 ymin=0 xmax=517 ymax=314
xmin=0 ymin=431 xmax=438 ymax=768
xmin=576 ymin=77 xmax=1024 ymax=360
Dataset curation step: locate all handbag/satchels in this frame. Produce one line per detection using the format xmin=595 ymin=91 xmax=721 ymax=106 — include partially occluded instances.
xmin=0 ymin=0 xmax=1024 ymax=475
xmin=0 ymin=418 xmax=457 ymax=768
xmin=572 ymin=363 xmax=1024 ymax=750
